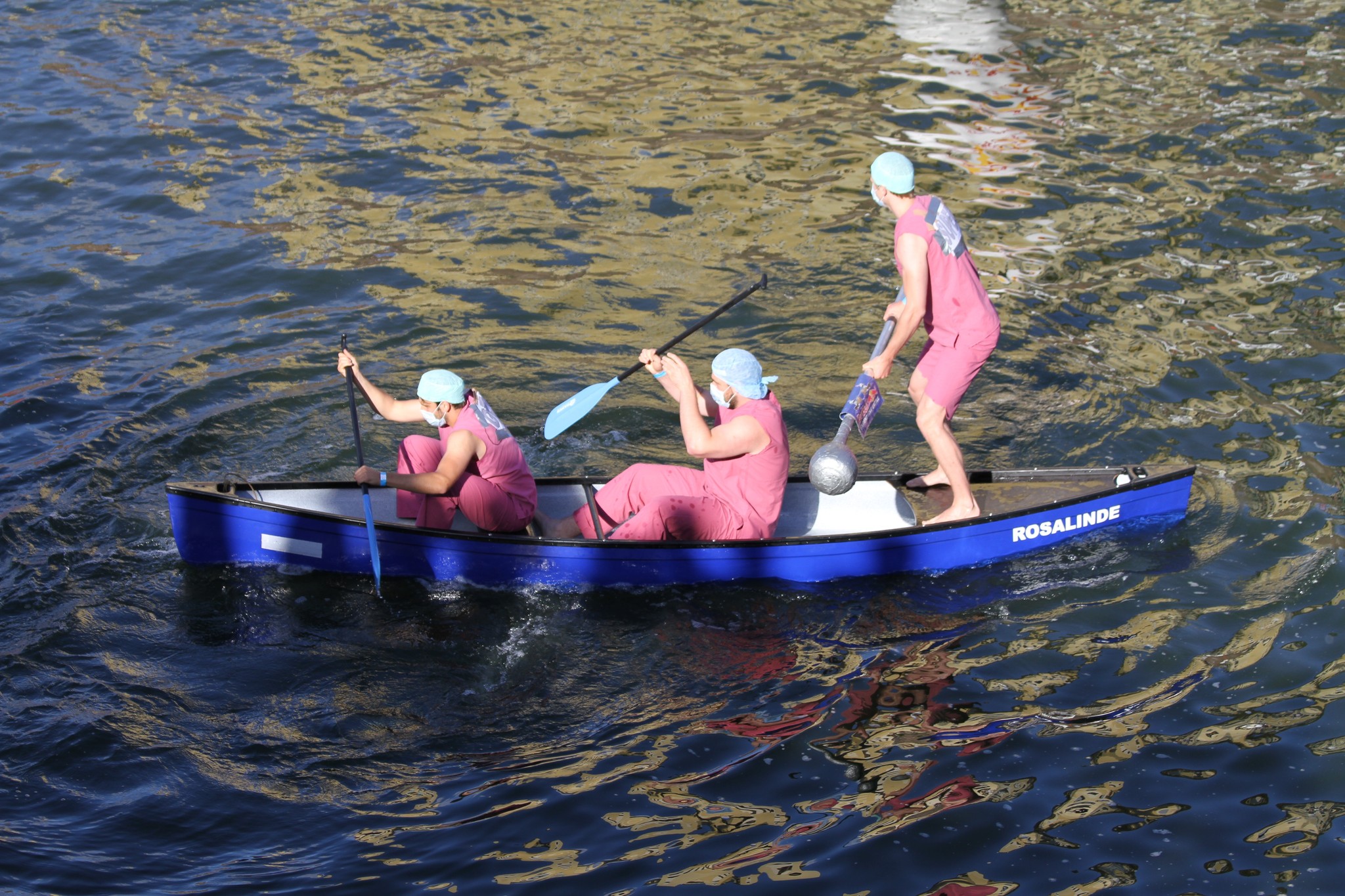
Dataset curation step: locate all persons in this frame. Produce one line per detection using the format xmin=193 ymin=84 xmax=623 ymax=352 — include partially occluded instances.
xmin=861 ymin=150 xmax=1001 ymax=525
xmin=534 ymin=347 xmax=791 ymax=540
xmin=337 ymin=348 xmax=537 ymax=535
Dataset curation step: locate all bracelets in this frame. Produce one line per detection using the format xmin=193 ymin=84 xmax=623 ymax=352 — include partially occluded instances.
xmin=652 ymin=370 xmax=668 ymax=378
xmin=380 ymin=470 xmax=387 ymax=487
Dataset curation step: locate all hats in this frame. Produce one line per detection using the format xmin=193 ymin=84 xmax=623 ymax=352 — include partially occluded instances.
xmin=711 ymin=348 xmax=779 ymax=399
xmin=416 ymin=369 xmax=466 ymax=404
xmin=871 ymin=152 xmax=916 ymax=194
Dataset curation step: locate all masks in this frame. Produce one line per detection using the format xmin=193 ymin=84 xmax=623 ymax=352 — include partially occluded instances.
xmin=710 ymin=381 xmax=736 ymax=408
xmin=419 ymin=400 xmax=447 ymax=427
xmin=871 ymin=183 xmax=888 ymax=208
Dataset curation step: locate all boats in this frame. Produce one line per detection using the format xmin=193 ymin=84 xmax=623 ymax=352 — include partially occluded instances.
xmin=165 ymin=461 xmax=1202 ymax=591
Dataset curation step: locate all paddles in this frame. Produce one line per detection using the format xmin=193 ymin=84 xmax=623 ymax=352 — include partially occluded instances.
xmin=544 ymin=274 xmax=768 ymax=440
xmin=341 ymin=332 xmax=380 ymax=595
